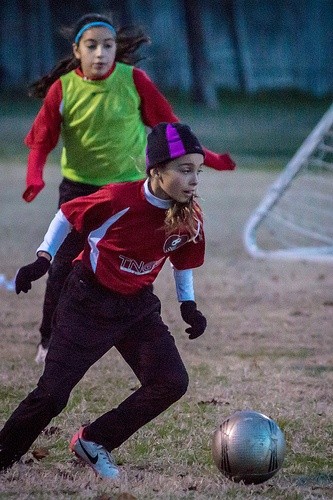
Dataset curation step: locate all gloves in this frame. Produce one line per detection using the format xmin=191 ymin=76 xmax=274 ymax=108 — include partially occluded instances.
xmin=179 ymin=300 xmax=207 ymax=340
xmin=15 ymin=256 xmax=51 ymax=295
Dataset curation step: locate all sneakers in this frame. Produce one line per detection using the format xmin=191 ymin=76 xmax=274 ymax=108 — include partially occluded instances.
xmin=70 ymin=424 xmax=121 ymax=484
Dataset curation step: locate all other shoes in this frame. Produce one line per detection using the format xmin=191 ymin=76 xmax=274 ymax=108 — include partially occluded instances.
xmin=35 ymin=344 xmax=49 ymax=366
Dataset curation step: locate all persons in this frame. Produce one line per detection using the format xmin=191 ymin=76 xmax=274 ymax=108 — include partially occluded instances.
xmin=0 ymin=123 xmax=207 ymax=482
xmin=22 ymin=14 xmax=235 ymax=364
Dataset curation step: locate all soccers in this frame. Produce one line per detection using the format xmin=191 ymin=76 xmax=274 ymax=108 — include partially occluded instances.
xmin=212 ymin=411 xmax=285 ymax=485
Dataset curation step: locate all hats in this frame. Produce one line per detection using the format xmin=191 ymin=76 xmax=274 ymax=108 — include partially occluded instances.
xmin=144 ymin=121 xmax=206 ymax=177
